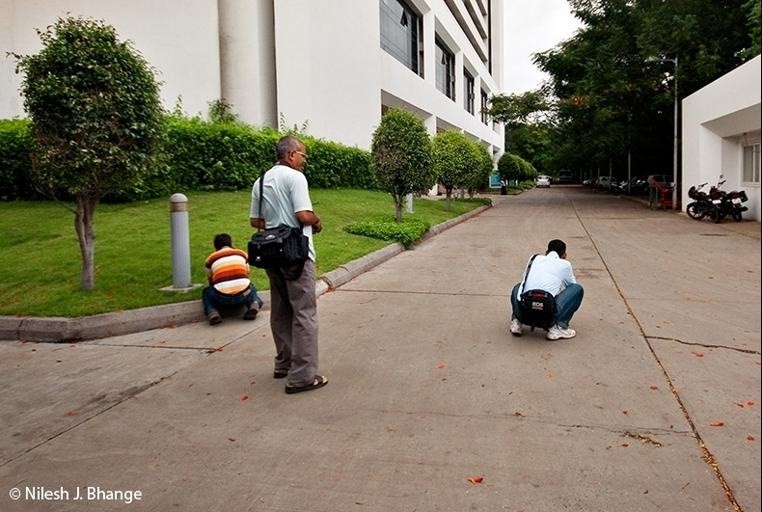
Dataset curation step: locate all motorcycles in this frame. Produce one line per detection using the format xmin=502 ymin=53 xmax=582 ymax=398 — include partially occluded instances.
xmin=686 ymin=183 xmax=727 ymax=222
xmin=709 ymin=173 xmax=749 ymax=223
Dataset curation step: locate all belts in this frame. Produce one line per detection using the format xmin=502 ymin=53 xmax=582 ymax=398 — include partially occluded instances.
xmin=214 ymin=286 xmax=250 ymax=298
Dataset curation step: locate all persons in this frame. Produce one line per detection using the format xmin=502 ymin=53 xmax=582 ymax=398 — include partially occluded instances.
xmin=510 ymin=239 xmax=586 ymax=339
xmin=201 ymin=233 xmax=262 ymax=325
xmin=250 ymin=136 xmax=328 ymax=395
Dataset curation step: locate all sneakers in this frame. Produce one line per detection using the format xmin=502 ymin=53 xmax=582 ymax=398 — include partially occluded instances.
xmin=509 ymin=319 xmax=522 ymax=336
xmin=243 ymin=302 xmax=259 ymax=320
xmin=546 ymin=324 xmax=576 ymax=340
xmin=207 ymin=310 xmax=222 ymax=325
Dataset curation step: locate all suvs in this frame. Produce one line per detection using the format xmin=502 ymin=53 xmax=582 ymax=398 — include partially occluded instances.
xmin=553 ymin=169 xmax=575 ymax=184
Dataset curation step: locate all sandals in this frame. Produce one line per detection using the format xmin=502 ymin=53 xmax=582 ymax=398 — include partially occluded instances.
xmin=285 ymin=375 xmax=328 ymax=394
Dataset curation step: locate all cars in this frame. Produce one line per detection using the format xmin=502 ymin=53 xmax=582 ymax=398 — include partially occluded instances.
xmin=535 ymin=174 xmax=552 ymax=189
xmin=581 ymin=174 xmax=673 ymax=195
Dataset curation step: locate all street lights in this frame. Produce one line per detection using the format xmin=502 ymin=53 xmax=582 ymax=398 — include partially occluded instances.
xmin=644 ymin=57 xmax=678 ymax=210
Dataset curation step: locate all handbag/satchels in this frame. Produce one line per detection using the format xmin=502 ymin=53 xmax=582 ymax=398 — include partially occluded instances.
xmin=517 ymin=289 xmax=558 ymax=328
xmin=248 ymin=224 xmax=309 ymax=269
